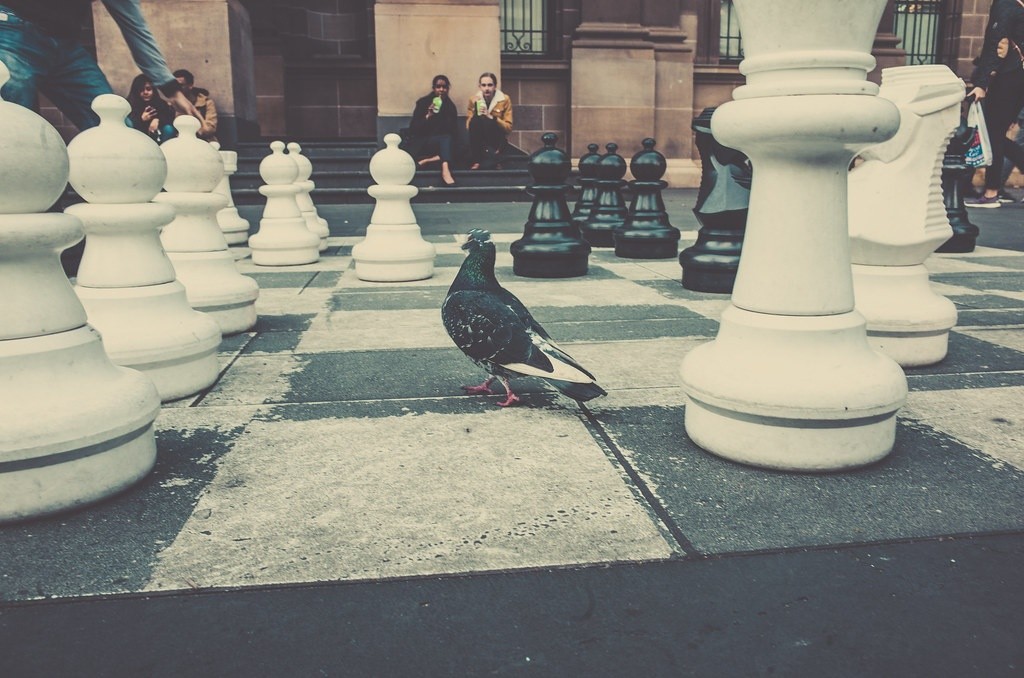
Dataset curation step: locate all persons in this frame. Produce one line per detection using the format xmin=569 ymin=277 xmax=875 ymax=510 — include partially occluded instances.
xmin=126 ymin=69 xmax=219 ymax=145
xmin=409 ymin=74 xmax=460 ymax=187
xmin=965 ymin=0 xmax=1024 ymax=211
xmin=466 ymin=72 xmax=513 ymax=170
xmin=0 ymin=0 xmax=208 ymax=136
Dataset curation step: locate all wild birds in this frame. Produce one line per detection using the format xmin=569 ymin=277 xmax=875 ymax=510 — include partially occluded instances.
xmin=441 ymin=229 xmax=608 ymax=408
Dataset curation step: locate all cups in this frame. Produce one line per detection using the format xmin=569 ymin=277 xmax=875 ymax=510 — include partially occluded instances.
xmin=433 ymin=97 xmax=442 ymax=113
xmin=477 ymin=100 xmax=485 ymax=116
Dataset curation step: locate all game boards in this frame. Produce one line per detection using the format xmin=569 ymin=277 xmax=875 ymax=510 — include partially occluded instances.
xmin=1 ymin=228 xmax=1024 ymax=604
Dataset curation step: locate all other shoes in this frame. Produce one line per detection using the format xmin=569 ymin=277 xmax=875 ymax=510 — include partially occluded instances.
xmin=415 ymin=159 xmax=423 ymax=170
xmin=440 ymin=175 xmax=457 ymax=187
